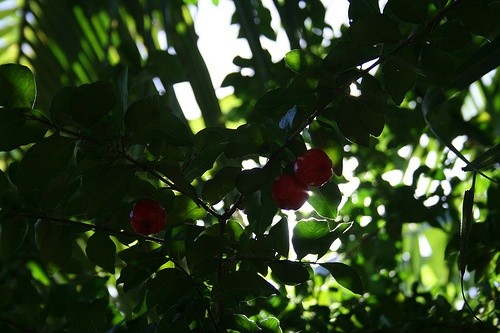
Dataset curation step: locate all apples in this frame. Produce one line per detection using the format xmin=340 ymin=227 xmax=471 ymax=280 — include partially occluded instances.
xmin=129 ymin=199 xmax=167 ymax=236
xmin=272 ymin=175 xmax=309 ymax=211
xmin=294 ymin=148 xmax=334 ymax=189
